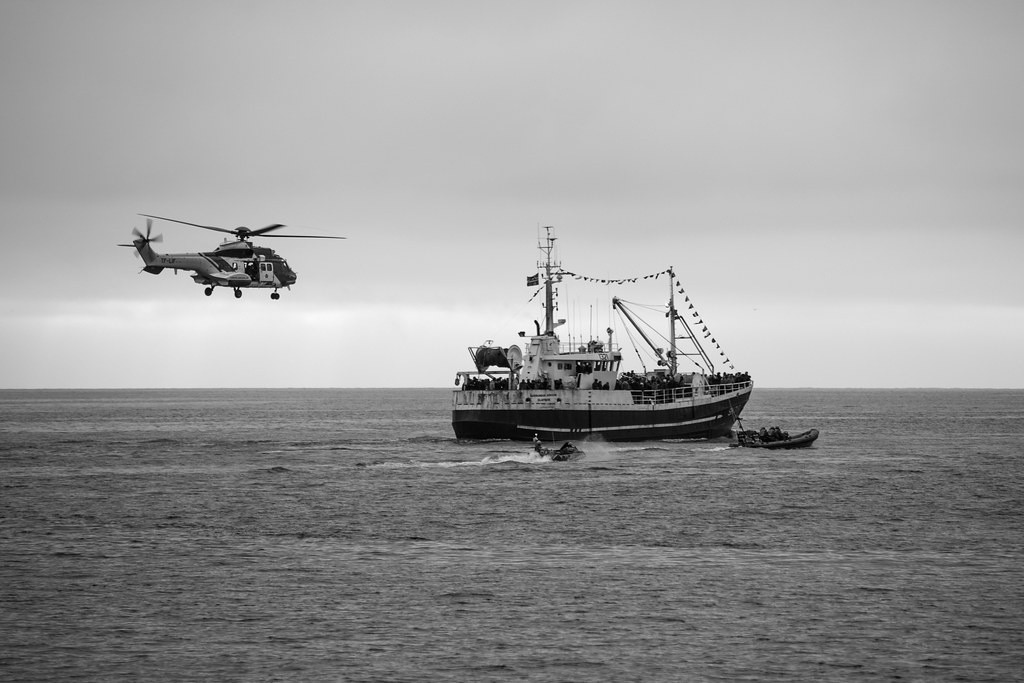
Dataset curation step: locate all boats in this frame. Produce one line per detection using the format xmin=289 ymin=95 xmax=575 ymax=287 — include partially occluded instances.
xmin=451 ymin=222 xmax=755 ymax=439
xmin=736 ymin=427 xmax=820 ymax=450
xmin=493 ymin=444 xmax=585 ymax=467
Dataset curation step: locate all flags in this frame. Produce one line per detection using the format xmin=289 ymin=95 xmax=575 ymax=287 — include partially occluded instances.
xmin=671 ymin=273 xmax=734 ymax=368
xmin=528 ymin=269 xmax=668 ymax=302
xmin=528 ymin=274 xmax=539 ymax=287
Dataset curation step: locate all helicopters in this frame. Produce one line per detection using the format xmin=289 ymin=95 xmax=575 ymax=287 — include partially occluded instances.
xmin=115 ymin=213 xmax=346 ymax=300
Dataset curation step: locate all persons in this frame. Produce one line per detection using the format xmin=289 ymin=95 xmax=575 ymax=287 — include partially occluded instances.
xmin=464 ymin=372 xmax=751 ymax=400
xmin=737 ymin=426 xmax=790 ymax=444
xmin=248 ymin=264 xmax=258 ymax=280
